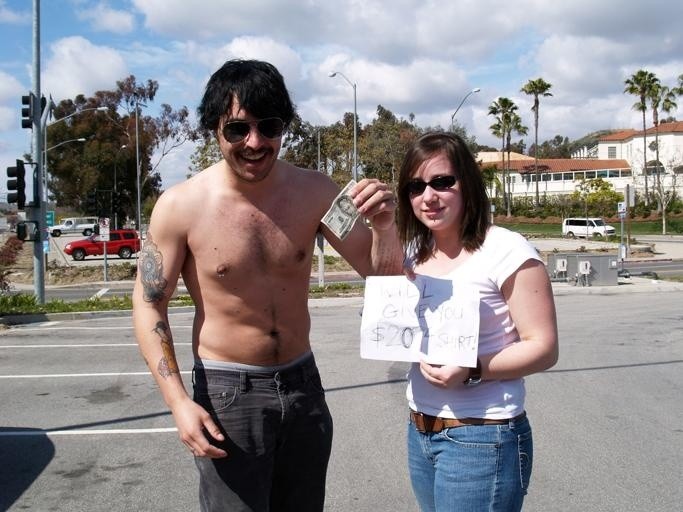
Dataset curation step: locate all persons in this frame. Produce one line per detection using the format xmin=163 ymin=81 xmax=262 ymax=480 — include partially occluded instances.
xmin=130 ymin=59 xmax=405 ymax=511
xmin=398 ymin=130 xmax=559 ymax=512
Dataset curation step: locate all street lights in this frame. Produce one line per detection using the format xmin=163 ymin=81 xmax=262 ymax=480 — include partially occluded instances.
xmin=451 ymin=88 xmax=480 ymax=132
xmin=328 ymin=70 xmax=358 ymax=182
xmin=42 ymin=107 xmax=110 ymax=214
xmin=135 ymin=102 xmax=146 ymax=240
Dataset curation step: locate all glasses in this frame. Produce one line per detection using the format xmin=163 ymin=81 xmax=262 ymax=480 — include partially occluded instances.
xmin=217 ymin=117 xmax=285 ymax=143
xmin=408 ymin=176 xmax=456 ymax=194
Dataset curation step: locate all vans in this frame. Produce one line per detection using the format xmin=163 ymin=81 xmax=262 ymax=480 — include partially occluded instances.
xmin=561 ymin=218 xmax=616 ymax=240
xmin=47 ymin=216 xmax=101 ymax=238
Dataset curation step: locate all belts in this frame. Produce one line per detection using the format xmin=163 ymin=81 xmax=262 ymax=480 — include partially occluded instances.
xmin=409 ymin=410 xmax=507 ymax=434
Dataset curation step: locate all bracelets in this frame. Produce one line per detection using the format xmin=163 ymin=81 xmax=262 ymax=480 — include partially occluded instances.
xmin=462 ymin=358 xmax=483 ymax=386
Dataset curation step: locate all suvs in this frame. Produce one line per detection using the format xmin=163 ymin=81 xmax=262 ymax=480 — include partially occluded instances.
xmin=63 ymin=229 xmax=140 ymax=260
